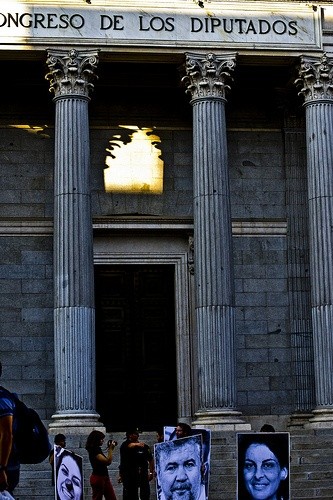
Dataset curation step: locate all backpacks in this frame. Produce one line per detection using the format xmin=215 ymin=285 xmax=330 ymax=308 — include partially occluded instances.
xmin=0 ymin=386 xmax=51 ymax=465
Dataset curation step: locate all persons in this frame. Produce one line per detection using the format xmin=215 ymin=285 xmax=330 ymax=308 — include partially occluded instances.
xmin=57 ymin=450 xmax=82 ymax=500
xmin=156 ymin=437 xmax=202 ymax=500
xmin=118 ymin=428 xmax=153 ymax=500
xmin=49 ymin=433 xmax=75 ymax=487
xmin=0 ymin=361 xmax=20 ymax=500
xmin=85 ymin=430 xmax=116 ymax=500
xmin=153 ymin=423 xmax=206 ymax=477
xmin=239 ymin=434 xmax=288 ymax=500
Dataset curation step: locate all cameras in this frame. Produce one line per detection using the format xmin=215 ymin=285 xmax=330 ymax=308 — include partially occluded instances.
xmin=108 ymin=440 xmax=117 ymax=447
